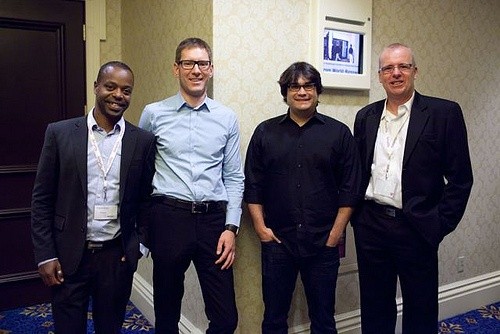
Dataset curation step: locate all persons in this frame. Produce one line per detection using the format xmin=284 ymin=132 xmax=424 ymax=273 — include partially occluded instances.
xmin=31 ymin=61 xmax=155 ymax=334
xmin=244 ymin=62 xmax=356 ymax=334
xmin=139 ymin=37 xmax=246 ymax=334
xmin=350 ymin=44 xmax=472 ymax=334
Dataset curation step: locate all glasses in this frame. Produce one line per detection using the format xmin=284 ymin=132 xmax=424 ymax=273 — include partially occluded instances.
xmin=285 ymin=83 xmax=316 ymax=91
xmin=380 ymin=63 xmax=414 ymax=74
xmin=177 ymin=59 xmax=211 ymax=71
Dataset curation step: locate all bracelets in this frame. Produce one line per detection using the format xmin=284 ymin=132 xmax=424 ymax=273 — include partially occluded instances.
xmin=224 ymin=225 xmax=239 ymax=238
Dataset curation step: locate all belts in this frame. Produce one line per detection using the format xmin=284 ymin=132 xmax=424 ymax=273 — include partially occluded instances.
xmin=365 ymin=200 xmax=403 ymax=218
xmin=159 ymin=196 xmax=226 ymax=215
xmin=82 ymin=238 xmax=121 ymax=252
